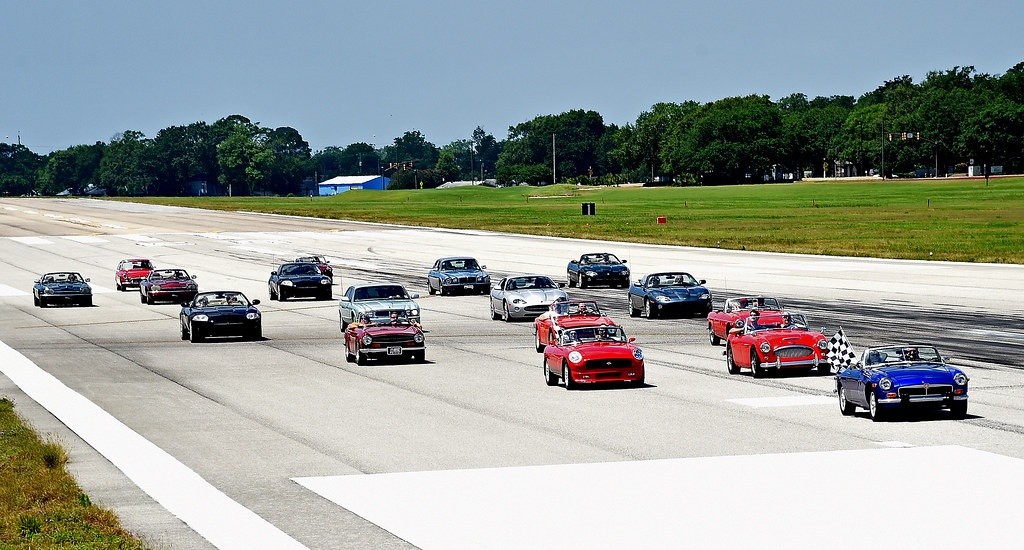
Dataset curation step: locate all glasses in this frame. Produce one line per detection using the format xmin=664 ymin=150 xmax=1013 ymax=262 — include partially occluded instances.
xmin=231 ymin=298 xmax=237 ymax=301
xmin=391 ymin=316 xmax=398 ymax=319
xmin=750 ymin=314 xmax=756 ymax=317
xmin=361 ymin=318 xmax=366 ymax=320
xmin=570 ymin=332 xmax=576 ymax=335
xmin=677 ymin=278 xmax=682 ymax=280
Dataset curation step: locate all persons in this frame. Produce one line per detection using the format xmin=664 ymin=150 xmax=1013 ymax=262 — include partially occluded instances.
xmin=737 ymin=310 xmax=766 ymax=336
xmin=781 ymin=312 xmax=799 ymax=329
xmin=647 ymin=277 xmax=660 ymax=288
xmin=738 ymin=299 xmax=749 ymax=310
xmin=223 ymin=295 xmax=242 ymax=305
xmin=345 ymin=314 xmax=377 ymax=328
xmin=386 ymin=312 xmax=403 ymax=325
xmin=864 ymin=350 xmax=882 ymax=366
xmin=900 ymin=345 xmax=927 ymax=364
xmin=574 ymin=302 xmax=591 ymax=314
xmin=673 ymin=275 xmax=689 ymax=285
xmin=755 ymin=298 xmax=766 ymax=309
xmin=581 ymin=256 xmax=592 ymax=264
xmin=596 ymin=325 xmax=611 ymax=341
xmin=196 ymin=295 xmax=210 ymax=308
xmin=600 ymin=254 xmax=612 ymax=264
xmin=567 ymin=329 xmax=582 ymax=343
xmin=535 ymin=304 xmax=569 ymax=325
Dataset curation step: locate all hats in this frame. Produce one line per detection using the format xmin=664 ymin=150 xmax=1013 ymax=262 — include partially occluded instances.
xmin=738 ymin=298 xmax=748 ymax=303
xmin=579 ymin=302 xmax=586 ymax=308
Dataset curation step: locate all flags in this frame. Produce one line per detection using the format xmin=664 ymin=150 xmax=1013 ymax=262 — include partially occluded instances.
xmin=825 ymin=330 xmax=859 ymax=374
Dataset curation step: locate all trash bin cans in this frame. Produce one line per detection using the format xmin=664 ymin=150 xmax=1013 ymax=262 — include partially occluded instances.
xmin=581 ymin=202 xmax=595 ymax=215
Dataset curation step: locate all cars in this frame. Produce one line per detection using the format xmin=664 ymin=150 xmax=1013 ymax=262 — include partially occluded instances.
xmin=269 ymin=253 xmax=335 ymax=301
xmin=115 ymin=259 xmax=155 ymax=292
xmin=138 ymin=269 xmax=199 ymax=303
xmin=567 ymin=253 xmax=631 ymax=290
xmin=534 ymin=299 xmax=616 ymax=354
xmin=707 ymin=296 xmax=785 ymax=347
xmin=721 ymin=314 xmax=829 ymax=378
xmin=428 ymin=256 xmax=491 ymax=296
xmin=33 ymin=271 xmax=93 ymax=308
xmin=833 ymin=344 xmax=971 ymax=422
xmin=338 ymin=282 xmax=420 ymax=332
xmin=178 ymin=290 xmax=263 ymax=343
xmin=344 ymin=320 xmax=425 ymax=366
xmin=489 ymin=275 xmax=570 ymax=321
xmin=628 ymin=271 xmax=713 ymax=321
xmin=542 ymin=326 xmax=645 ymax=390
